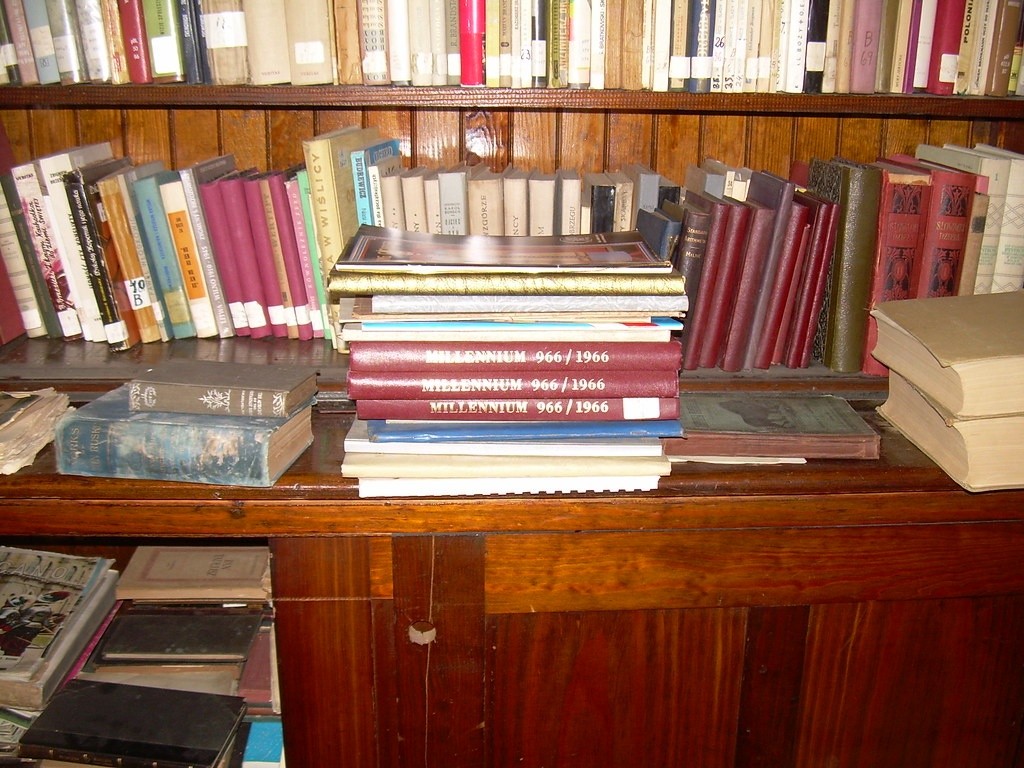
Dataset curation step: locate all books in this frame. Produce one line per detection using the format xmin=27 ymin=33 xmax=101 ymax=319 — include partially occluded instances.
xmin=0 ymin=126 xmax=1023 ymax=499
xmin=0 ymin=543 xmax=287 ymax=768
xmin=0 ymin=0 xmax=1024 ymax=96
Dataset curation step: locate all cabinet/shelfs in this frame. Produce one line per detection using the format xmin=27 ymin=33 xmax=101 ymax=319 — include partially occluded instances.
xmin=0 ymin=0 xmax=1024 ymax=768
